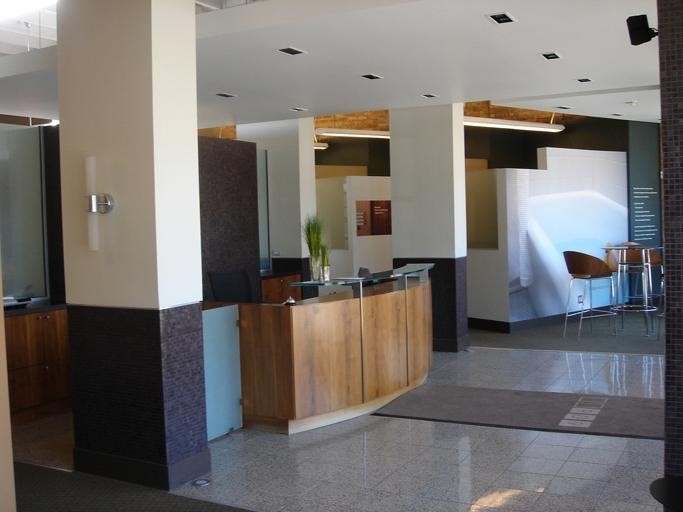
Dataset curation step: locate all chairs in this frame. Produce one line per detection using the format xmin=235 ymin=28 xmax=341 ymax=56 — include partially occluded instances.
xmin=561 ymin=242 xmax=666 ymax=340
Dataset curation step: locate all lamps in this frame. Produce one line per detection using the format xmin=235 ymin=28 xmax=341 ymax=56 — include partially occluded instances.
xmin=625 ymin=14 xmax=659 ymax=44
xmin=316 ymin=127 xmax=391 ymax=141
xmin=464 ymin=108 xmax=567 ymax=134
xmin=81 ymin=154 xmax=114 ymax=251
xmin=314 ymin=135 xmax=329 ymax=149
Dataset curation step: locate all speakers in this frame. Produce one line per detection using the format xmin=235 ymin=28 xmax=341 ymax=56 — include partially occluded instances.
xmin=625 ymin=13 xmax=651 ymax=45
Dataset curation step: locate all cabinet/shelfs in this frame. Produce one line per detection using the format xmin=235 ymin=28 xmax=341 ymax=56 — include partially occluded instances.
xmin=4 ymin=309 xmax=70 ymax=431
xmin=260 ymin=274 xmax=302 ymax=303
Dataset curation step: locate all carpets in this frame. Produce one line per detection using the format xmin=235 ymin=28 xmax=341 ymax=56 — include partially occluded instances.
xmin=13 ymin=458 xmax=253 ymax=512
xmin=372 ymin=382 xmax=665 ymax=439
xmin=466 ymin=304 xmax=666 ymax=356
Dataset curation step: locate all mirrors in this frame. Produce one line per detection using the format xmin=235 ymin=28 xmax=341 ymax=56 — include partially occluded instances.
xmin=0 ymin=122 xmax=50 ymax=308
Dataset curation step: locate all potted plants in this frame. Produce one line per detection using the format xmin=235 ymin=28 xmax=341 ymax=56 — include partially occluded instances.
xmin=300 ymin=215 xmax=325 ymax=282
xmin=321 ymin=245 xmax=331 ymax=281
